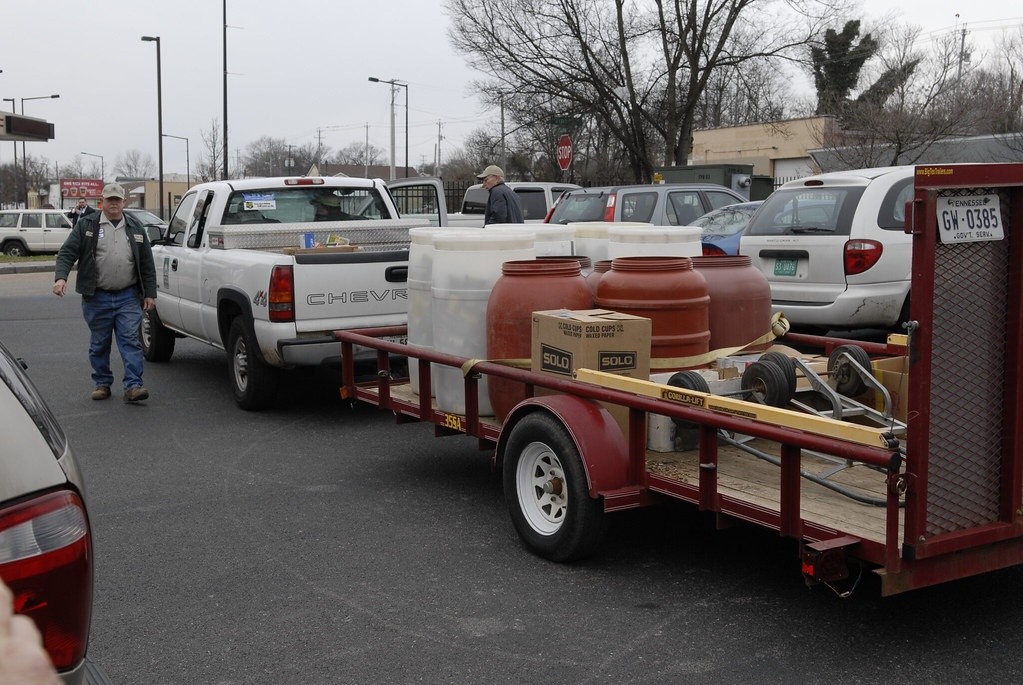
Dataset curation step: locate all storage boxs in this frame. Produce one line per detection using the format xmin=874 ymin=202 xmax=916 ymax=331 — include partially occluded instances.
xmin=284 ymin=244 xmax=358 ymax=258
xmin=532 ymin=309 xmax=907 ymax=452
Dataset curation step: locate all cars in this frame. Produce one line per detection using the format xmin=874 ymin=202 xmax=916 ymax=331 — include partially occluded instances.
xmin=742 ymin=165 xmax=918 ymax=327
xmin=459 ymin=179 xmax=763 ymax=254
xmin=0 ymin=345 xmax=95 ymax=682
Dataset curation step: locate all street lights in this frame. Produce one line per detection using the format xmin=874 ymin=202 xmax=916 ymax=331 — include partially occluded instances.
xmin=20 ymin=94 xmax=60 ymax=208
xmin=142 ymin=33 xmax=162 ymax=219
xmin=161 ymin=134 xmax=191 ymax=193
xmin=77 ymin=150 xmax=106 ymax=186
xmin=369 ymin=74 xmax=398 ymax=181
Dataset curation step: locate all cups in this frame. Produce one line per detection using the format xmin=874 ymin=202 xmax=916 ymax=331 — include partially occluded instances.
xmin=305 ymin=233 xmax=314 ymax=248
xmin=300 ymin=235 xmax=305 ymax=249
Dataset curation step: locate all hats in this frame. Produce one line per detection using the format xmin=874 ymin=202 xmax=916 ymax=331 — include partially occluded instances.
xmin=477 ymin=165 xmax=505 ymax=178
xmin=102 ymin=184 xmax=125 ymax=199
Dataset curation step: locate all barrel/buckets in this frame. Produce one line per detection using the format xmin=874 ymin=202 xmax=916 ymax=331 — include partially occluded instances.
xmin=407 ymin=220 xmax=772 ymax=424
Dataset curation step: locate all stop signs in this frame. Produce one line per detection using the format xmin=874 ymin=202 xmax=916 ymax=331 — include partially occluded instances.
xmin=556 ymin=134 xmax=574 ymax=170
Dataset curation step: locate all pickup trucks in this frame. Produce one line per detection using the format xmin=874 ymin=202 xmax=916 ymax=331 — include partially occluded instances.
xmin=135 ymin=174 xmax=431 ymax=413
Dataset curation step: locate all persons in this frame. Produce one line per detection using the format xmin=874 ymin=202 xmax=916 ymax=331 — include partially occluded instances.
xmin=67 ymin=198 xmax=95 ymax=229
xmin=476 ymin=165 xmax=524 ymax=228
xmin=679 ymin=204 xmax=697 ymax=226
xmin=310 ymin=194 xmax=342 ymax=221
xmin=54 ymin=184 xmax=157 ymax=402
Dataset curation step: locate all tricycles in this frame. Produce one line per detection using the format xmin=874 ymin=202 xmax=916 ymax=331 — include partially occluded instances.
xmin=341 ymin=158 xmax=1023 ymax=607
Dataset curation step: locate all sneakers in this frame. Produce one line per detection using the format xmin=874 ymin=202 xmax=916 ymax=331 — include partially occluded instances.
xmin=92 ymin=386 xmax=111 ymax=399
xmin=123 ymin=387 xmax=149 ymax=402
